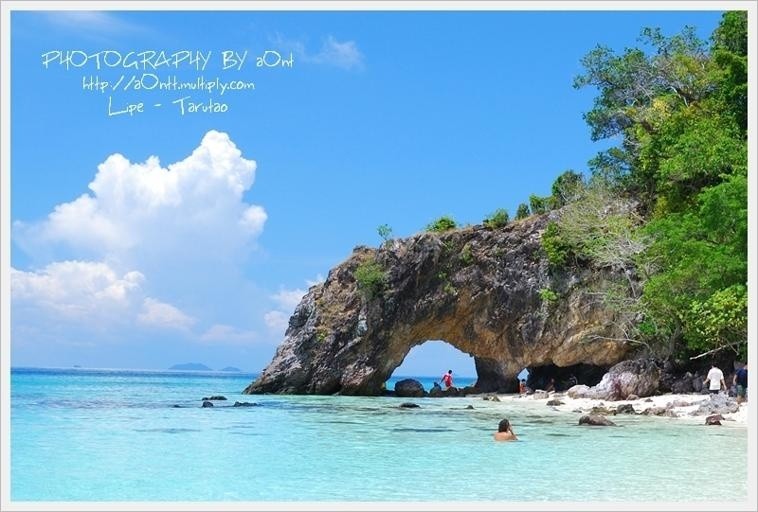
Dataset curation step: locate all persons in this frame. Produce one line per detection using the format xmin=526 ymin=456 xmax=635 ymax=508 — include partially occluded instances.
xmin=703 ymin=360 xmax=727 ymax=395
xmin=543 ymin=378 xmax=560 ymax=393
xmin=493 ymin=419 xmax=518 ymax=442
xmin=439 ymin=370 xmax=454 ymax=389
xmin=564 ymin=373 xmax=578 ymax=389
xmin=519 ymin=379 xmax=527 ymax=397
xmin=733 ymin=361 xmax=748 ymax=405
